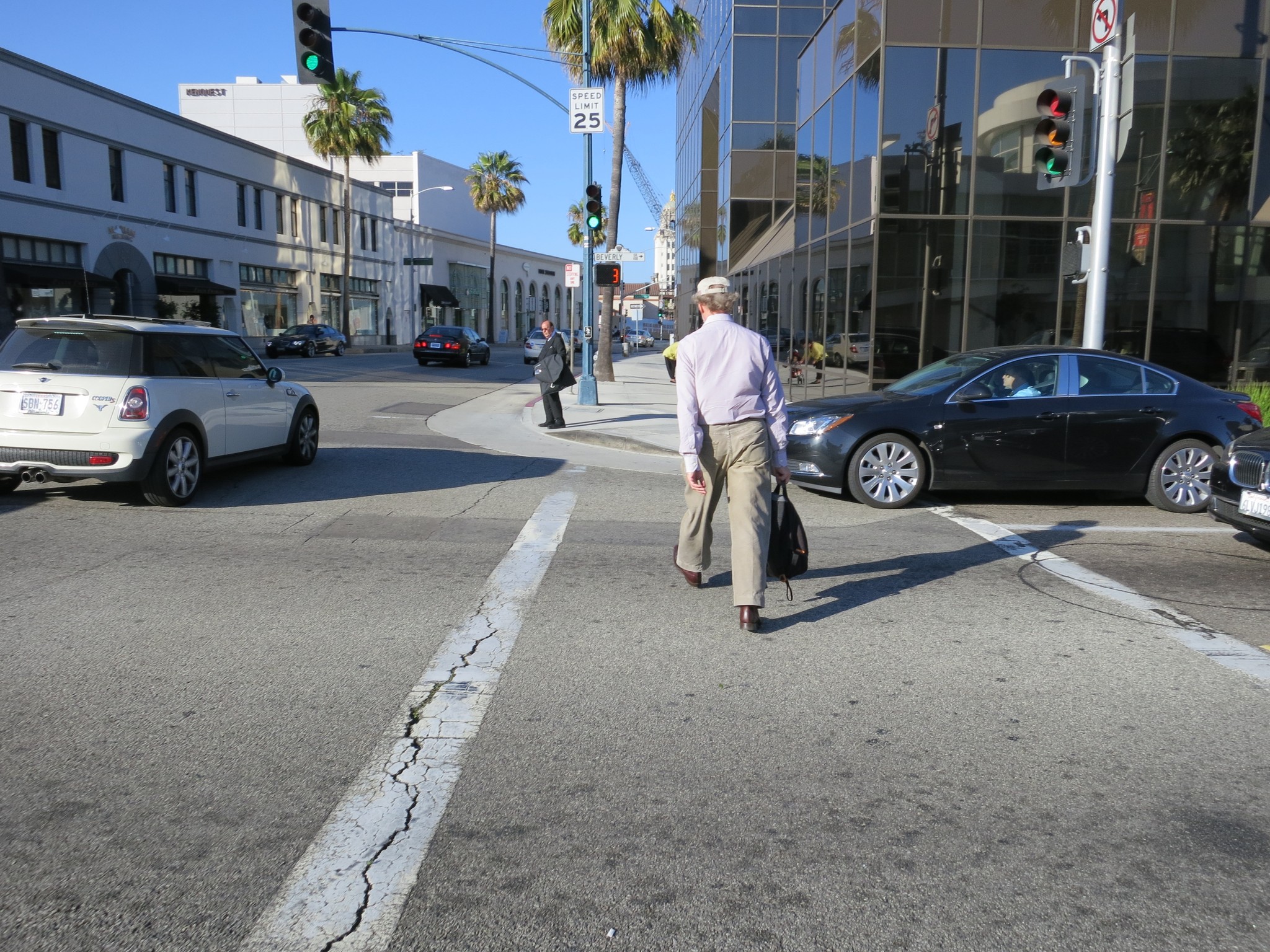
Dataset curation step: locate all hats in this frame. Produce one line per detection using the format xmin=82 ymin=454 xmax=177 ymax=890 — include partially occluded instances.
xmin=697 ymin=276 xmax=734 ymax=295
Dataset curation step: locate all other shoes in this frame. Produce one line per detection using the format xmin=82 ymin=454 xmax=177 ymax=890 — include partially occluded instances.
xmin=670 ymin=380 xmax=675 ymax=383
xmin=811 ymin=381 xmax=820 ymax=384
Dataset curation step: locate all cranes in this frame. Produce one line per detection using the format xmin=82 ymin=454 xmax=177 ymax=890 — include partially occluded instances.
xmin=604 ymin=119 xmax=676 ymax=252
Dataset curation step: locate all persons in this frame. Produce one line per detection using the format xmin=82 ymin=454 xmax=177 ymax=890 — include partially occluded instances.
xmin=308 ymin=315 xmax=317 ymax=325
xmin=663 ymin=340 xmax=680 ymax=383
xmin=792 ymin=355 xmax=801 ymax=377
xmin=670 ymin=275 xmax=793 ymax=630
xmin=799 ymin=340 xmax=826 ymax=384
xmin=999 ymin=361 xmax=1040 ymax=398
xmin=536 ymin=321 xmax=573 ymax=429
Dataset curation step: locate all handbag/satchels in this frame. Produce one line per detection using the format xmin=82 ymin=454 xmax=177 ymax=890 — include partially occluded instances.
xmin=766 ymin=476 xmax=808 ymax=601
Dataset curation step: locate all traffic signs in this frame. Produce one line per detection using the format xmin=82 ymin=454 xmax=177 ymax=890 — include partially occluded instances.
xmin=630 ymin=303 xmax=645 ymax=310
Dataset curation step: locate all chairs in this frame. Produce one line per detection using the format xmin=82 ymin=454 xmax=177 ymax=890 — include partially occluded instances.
xmin=1122 ymin=374 xmax=1155 ymax=395
xmin=1086 ymin=372 xmax=1112 ymax=395
xmin=1037 ymin=371 xmax=1057 ymax=399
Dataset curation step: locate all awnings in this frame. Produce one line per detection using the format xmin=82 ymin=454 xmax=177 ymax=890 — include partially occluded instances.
xmin=0 ymin=262 xmax=125 ymax=289
xmin=155 ymin=275 xmax=236 ymax=295
xmin=419 ymin=283 xmax=460 ymax=307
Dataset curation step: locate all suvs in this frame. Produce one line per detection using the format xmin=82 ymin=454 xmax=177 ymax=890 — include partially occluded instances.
xmin=0 ymin=315 xmax=320 ymax=506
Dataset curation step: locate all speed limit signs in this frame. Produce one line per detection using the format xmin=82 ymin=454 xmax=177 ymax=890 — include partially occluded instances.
xmin=568 ymin=87 xmax=605 ymax=134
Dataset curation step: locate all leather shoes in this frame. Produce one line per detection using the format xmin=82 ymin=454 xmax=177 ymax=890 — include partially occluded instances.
xmin=547 ymin=419 xmax=565 ymax=429
xmin=674 ymin=544 xmax=701 ymax=587
xmin=538 ymin=418 xmax=554 ymax=427
xmin=739 ymin=605 xmax=761 ymax=630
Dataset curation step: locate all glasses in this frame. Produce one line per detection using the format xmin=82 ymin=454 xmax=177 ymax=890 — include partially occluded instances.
xmin=542 ymin=327 xmax=551 ymax=331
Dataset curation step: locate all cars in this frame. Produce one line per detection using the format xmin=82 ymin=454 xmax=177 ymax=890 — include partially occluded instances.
xmin=524 ymin=326 xmax=541 ymax=344
xmin=523 ymin=331 xmax=576 ymax=365
xmin=413 ymin=325 xmax=490 ymax=369
xmin=1207 ymin=425 xmax=1270 ymax=551
xmin=625 ymin=329 xmax=655 ymax=347
xmin=770 ymin=343 xmax=1263 ymax=514
xmin=560 ymin=329 xmax=583 ymax=353
xmin=620 ymin=329 xmax=632 ymax=343
xmin=266 ymin=324 xmax=347 ymax=359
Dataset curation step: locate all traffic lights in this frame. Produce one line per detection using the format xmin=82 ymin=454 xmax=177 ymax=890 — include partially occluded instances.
xmin=658 ymin=309 xmax=662 ymax=317
xmin=1034 ymin=73 xmax=1086 ymax=193
xmin=291 ymin=0 xmax=336 ymax=85
xmin=586 ymin=185 xmax=602 ymax=231
xmin=622 ymin=282 xmax=625 ymax=290
xmin=593 ymin=262 xmax=621 ymax=286
xmin=625 ymin=309 xmax=628 ymax=317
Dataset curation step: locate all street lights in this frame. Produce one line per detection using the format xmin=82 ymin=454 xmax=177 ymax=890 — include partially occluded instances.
xmin=1127 ymin=131 xmax=1175 ymax=255
xmin=410 ymin=186 xmax=456 ymax=346
xmin=660 ymin=273 xmax=676 ymax=342
